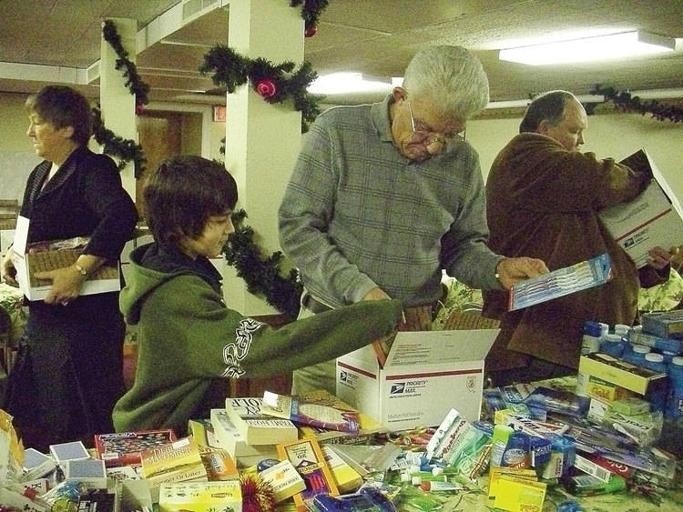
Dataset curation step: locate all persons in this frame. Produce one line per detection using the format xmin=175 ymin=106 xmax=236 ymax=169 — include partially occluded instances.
xmin=112 ymin=155 xmax=402 ymax=433
xmin=278 ymin=45 xmax=550 ymax=394
xmin=479 ymin=90 xmax=679 ymax=386
xmin=0 ymin=85 xmax=137 ymax=452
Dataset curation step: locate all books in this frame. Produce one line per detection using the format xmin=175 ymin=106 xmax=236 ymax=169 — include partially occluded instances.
xmin=23 ymin=391 xmax=369 ymax=512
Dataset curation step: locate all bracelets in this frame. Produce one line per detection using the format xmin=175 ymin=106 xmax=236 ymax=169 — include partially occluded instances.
xmin=495 ymin=258 xmax=507 ymax=279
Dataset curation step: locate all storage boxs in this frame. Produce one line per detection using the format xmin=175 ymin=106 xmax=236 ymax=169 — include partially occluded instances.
xmin=596 ymin=147 xmax=681 ymax=272
xmin=8 ymin=239 xmax=123 ymax=301
xmin=334 ymin=310 xmax=502 ymax=438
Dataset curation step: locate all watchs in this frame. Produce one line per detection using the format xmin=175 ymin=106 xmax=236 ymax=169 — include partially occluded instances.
xmin=74 ymin=263 xmax=89 ymax=280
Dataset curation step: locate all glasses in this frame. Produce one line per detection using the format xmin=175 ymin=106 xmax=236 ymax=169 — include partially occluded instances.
xmin=407 ymin=102 xmax=469 ymax=146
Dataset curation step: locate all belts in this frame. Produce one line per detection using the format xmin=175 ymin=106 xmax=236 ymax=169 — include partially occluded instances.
xmin=302 ymin=292 xmax=333 ymax=315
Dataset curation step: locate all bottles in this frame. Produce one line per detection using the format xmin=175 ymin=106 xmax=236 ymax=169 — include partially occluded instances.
xmin=488 ymin=425 xmax=551 ymax=475
xmin=578 ymin=320 xmax=683 ymax=420
